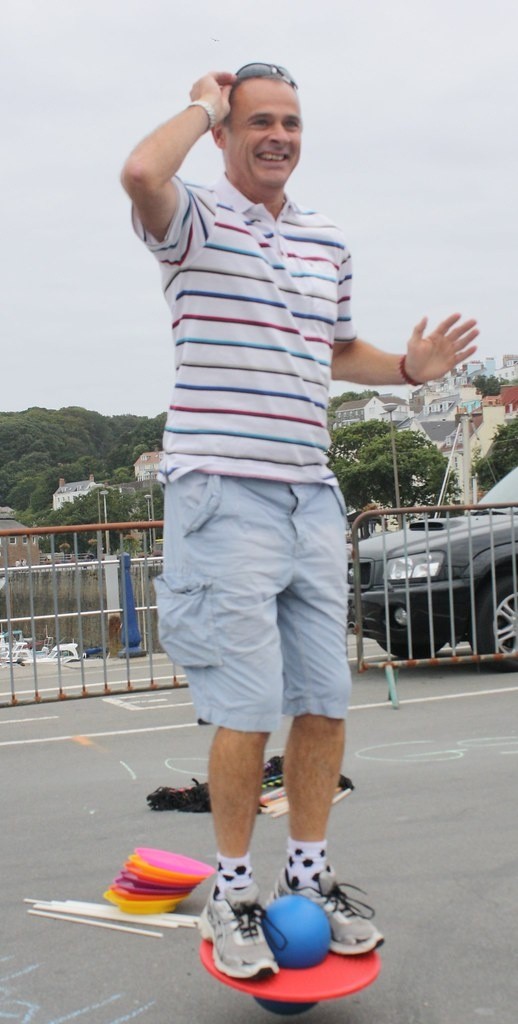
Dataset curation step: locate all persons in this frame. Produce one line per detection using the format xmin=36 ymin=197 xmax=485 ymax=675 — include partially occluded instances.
xmin=121 ymin=63 xmax=479 ymax=979
xmin=15 ymin=558 xmax=26 ymax=567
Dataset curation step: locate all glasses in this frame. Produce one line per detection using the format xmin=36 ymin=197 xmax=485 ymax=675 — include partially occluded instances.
xmin=231 ymin=63 xmax=298 ymax=91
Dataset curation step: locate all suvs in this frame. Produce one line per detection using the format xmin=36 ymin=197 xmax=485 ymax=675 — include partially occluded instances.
xmin=344 ymin=464 xmax=518 ymax=671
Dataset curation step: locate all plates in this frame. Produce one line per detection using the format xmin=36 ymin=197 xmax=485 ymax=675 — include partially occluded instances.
xmin=103 ymin=847 xmax=216 ymax=915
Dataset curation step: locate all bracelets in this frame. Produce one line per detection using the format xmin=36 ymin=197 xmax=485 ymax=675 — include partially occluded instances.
xmin=399 ymin=355 xmax=424 ymax=386
xmin=187 ymin=101 xmax=216 ymax=132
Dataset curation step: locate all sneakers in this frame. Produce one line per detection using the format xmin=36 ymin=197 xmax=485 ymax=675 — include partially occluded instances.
xmin=266 ymin=865 xmax=385 ymax=955
xmin=198 ymin=880 xmax=279 ymax=980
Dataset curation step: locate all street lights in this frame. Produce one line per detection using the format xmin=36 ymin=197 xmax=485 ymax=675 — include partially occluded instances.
xmin=382 ymin=401 xmax=403 ymax=530
xmin=144 ymin=495 xmax=153 ymax=552
xmin=100 ymin=491 xmax=109 ymax=553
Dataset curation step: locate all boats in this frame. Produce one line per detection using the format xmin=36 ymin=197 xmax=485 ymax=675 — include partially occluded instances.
xmin=0 ymin=629 xmax=111 ymax=664
